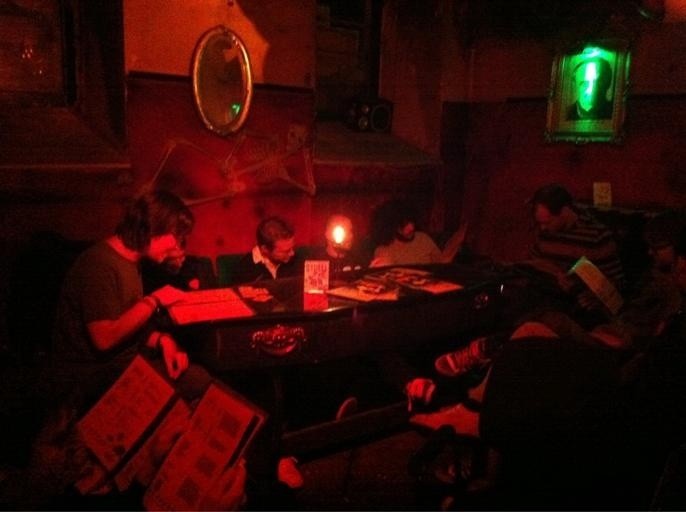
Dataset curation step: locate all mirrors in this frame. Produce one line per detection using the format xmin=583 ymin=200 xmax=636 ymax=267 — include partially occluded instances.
xmin=190 ymin=25 xmax=255 ymax=138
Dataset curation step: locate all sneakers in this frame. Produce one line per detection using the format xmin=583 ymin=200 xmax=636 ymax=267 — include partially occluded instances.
xmin=278 ymin=463 xmax=304 ymax=489
xmin=336 ymin=397 xmax=358 ymax=420
xmin=409 ymin=403 xmax=481 ymax=437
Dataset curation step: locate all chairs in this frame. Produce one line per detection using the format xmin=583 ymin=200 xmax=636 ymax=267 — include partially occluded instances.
xmin=31 ymin=241 xmax=684 ymax=512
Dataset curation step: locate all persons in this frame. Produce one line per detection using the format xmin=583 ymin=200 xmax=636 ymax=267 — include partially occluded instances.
xmin=563 ymin=54 xmax=613 ymax=122
xmin=0 ymin=180 xmax=686 ymax=512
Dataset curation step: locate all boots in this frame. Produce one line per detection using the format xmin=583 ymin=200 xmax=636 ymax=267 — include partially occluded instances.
xmin=435 ymin=457 xmax=490 ymax=491
xmin=434 ymin=339 xmax=492 ymax=377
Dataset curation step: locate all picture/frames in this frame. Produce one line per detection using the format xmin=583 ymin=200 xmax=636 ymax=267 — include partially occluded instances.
xmin=541 ymin=11 xmax=634 ymax=145
